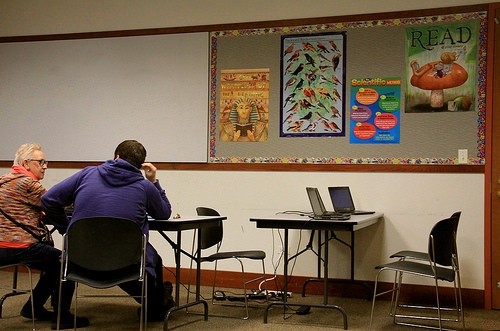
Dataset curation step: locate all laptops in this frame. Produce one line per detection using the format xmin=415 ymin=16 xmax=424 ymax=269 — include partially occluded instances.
xmin=306 ymin=187 xmax=351 ymax=220
xmin=328 ymin=186 xmax=375 ymax=215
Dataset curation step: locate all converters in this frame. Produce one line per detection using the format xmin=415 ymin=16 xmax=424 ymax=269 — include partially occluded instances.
xmin=296 ymin=306 xmax=310 ymax=315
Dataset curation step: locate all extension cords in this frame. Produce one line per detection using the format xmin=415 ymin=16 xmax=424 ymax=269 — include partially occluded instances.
xmin=260 ymin=290 xmax=294 ymax=297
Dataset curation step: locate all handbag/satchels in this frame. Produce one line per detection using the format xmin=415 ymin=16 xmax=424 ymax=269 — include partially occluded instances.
xmin=40 ymin=226 xmax=54 ymax=246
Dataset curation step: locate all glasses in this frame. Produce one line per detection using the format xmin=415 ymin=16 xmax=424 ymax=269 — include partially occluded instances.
xmin=27 ymin=158 xmax=48 ymax=166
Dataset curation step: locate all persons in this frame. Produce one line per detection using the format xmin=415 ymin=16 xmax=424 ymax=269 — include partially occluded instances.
xmin=0 ymin=143 xmax=89 ymax=330
xmin=41 ymin=140 xmax=173 ymax=321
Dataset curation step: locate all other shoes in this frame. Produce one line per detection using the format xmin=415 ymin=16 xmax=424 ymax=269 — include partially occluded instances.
xmin=163 ymin=281 xmax=173 ymax=297
xmin=19 ymin=302 xmax=53 ymax=320
xmin=50 ymin=312 xmax=90 ymax=330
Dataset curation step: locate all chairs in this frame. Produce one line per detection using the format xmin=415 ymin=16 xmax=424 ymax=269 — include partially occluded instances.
xmin=0 ymin=241 xmax=53 ymax=330
xmin=56 ymin=215 xmax=150 ymax=331
xmin=370 ymin=219 xmax=466 ymax=331
xmin=389 ymin=211 xmax=464 ymax=321
xmin=196 ymin=206 xmax=269 ymax=319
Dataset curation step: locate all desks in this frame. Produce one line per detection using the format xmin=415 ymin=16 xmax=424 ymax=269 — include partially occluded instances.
xmin=118 ymin=216 xmax=228 ymax=331
xmin=250 ymin=211 xmax=385 ymax=330
